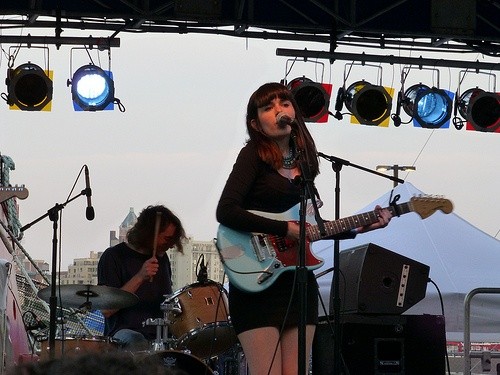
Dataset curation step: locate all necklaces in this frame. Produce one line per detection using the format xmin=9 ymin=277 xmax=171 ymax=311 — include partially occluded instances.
xmin=281 ymin=134 xmax=300 ymax=169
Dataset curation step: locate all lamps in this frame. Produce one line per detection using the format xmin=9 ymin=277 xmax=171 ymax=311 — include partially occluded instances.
xmin=335 ymin=63 xmax=395 ymax=128
xmin=454 ymin=70 xmax=500 ymax=133
xmin=67 ymin=47 xmax=126 ymax=113
xmin=280 ymin=59 xmax=335 ymax=123
xmin=392 ymin=67 xmax=454 ymax=128
xmin=1 ymin=46 xmax=54 ymax=111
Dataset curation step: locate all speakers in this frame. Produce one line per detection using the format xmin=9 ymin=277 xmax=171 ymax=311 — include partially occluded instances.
xmin=312 ymin=316 xmax=446 ymax=375
xmin=329 ymin=242 xmax=430 ymax=316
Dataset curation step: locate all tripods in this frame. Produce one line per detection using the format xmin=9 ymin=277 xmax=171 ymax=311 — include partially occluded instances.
xmin=267 ymin=130 xmax=350 ymax=375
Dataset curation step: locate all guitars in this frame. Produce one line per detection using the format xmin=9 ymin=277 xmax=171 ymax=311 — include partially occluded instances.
xmin=0 ymin=183 xmax=29 ymax=205
xmin=216 ymin=193 xmax=453 ymax=293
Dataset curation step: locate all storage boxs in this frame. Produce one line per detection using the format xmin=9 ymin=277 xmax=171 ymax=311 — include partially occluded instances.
xmin=312 ymin=313 xmax=446 ymax=375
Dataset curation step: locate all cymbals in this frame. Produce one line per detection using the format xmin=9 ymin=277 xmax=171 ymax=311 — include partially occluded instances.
xmin=37 ymin=284 xmax=139 ymax=310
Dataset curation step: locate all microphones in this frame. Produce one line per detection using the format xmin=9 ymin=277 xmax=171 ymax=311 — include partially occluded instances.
xmin=277 ymin=112 xmax=296 ymax=130
xmin=85 ymin=166 xmax=95 ymax=220
xmin=197 ymin=257 xmax=207 ymax=282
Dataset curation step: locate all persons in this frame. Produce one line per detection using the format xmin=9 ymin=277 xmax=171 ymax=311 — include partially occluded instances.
xmin=216 ymin=83 xmax=392 ymax=375
xmin=98 ymin=206 xmax=184 ymax=337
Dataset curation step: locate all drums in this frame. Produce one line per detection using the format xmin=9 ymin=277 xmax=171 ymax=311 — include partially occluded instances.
xmin=139 ymin=350 xmax=219 ymax=375
xmin=40 ymin=336 xmax=120 ymax=360
xmin=159 ymin=279 xmax=238 ymax=360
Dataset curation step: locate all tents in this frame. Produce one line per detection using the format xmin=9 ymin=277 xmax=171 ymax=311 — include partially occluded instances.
xmin=222 ymin=182 xmax=500 ymax=333
